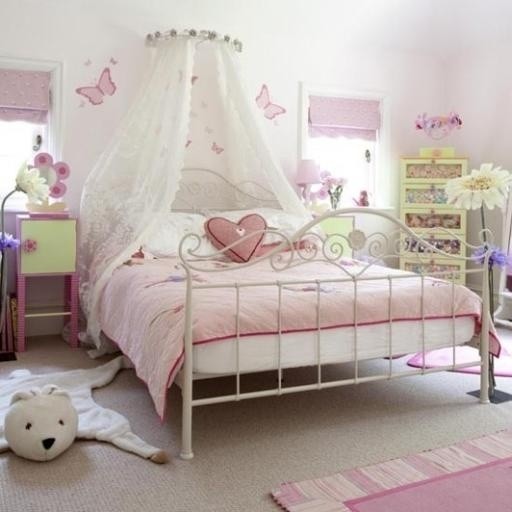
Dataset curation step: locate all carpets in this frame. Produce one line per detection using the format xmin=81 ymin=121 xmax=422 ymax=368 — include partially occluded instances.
xmin=267 ymin=425 xmax=511 ymax=511
xmin=406 ymin=336 xmax=512 ymax=376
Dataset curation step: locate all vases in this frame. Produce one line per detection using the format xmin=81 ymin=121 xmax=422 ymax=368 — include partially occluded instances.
xmin=329 ymin=192 xmax=342 ymax=211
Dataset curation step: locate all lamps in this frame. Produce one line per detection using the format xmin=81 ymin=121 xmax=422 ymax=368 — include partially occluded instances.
xmin=295 ymin=158 xmax=321 ymax=208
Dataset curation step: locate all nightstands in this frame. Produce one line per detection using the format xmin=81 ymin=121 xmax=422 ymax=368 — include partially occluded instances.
xmin=311 ymin=212 xmax=356 ymax=261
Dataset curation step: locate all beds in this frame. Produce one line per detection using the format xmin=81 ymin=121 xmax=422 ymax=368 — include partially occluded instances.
xmin=86 ymin=170 xmax=496 ymax=461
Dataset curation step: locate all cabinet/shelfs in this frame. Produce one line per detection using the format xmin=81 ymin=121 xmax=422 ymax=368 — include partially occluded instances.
xmin=17 ymin=213 xmax=79 ymax=353
xmin=400 ymin=159 xmax=467 ymax=285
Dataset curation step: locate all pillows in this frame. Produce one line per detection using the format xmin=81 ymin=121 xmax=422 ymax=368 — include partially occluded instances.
xmin=120 ymin=206 xmax=312 ymax=263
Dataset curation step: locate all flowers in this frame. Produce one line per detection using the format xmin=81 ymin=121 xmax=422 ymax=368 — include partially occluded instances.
xmin=321 ymin=176 xmax=347 ymax=210
